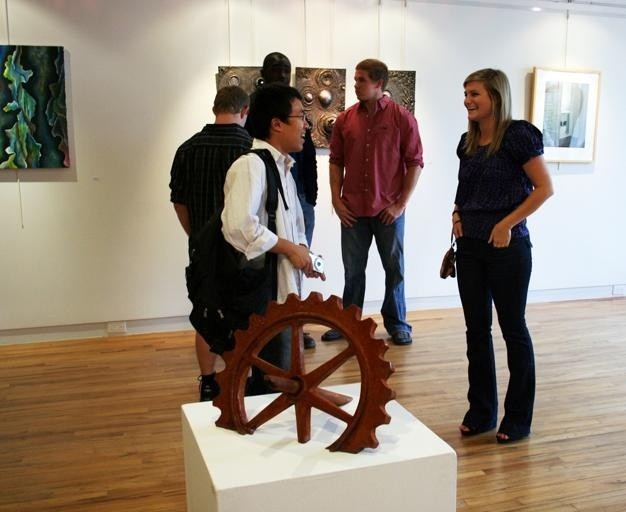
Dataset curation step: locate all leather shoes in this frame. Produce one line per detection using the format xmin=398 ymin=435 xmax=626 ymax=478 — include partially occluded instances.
xmin=201 ymin=371 xmax=220 ymax=401
xmin=392 ymin=331 xmax=412 ymax=345
xmin=303 ymin=328 xmax=343 ymax=348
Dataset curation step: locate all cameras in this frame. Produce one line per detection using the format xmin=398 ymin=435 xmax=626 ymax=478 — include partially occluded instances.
xmin=309 ymin=252 xmax=325 ymax=274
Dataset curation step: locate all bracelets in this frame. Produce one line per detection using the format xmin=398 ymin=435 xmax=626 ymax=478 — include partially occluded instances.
xmin=451 ymin=210 xmax=460 ymax=215
xmin=453 ymin=219 xmax=463 ymax=224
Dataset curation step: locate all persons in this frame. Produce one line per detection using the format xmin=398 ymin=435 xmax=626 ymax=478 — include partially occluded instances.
xmin=244 ymin=52 xmax=319 ymax=348
xmin=167 ymin=86 xmax=254 ymax=401
xmin=320 ymin=59 xmax=426 ymax=348
xmin=450 ymin=66 xmax=555 ymax=448
xmin=214 ymin=80 xmax=327 ymax=395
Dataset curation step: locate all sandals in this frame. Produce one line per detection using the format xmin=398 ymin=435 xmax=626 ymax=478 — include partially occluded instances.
xmin=461 ymin=419 xmax=497 ymax=436
xmin=496 ymin=428 xmax=530 ymax=443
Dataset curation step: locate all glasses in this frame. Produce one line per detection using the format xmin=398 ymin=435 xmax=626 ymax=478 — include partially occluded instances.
xmin=288 ymin=113 xmax=306 ymax=122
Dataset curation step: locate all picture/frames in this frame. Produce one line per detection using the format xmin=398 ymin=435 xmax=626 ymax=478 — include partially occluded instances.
xmin=528 ymin=65 xmax=602 ymax=165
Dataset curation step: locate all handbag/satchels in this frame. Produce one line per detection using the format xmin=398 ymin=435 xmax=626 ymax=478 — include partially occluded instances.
xmin=440 ymin=247 xmax=457 ymax=278
xmin=186 ymin=212 xmax=289 ymax=353
